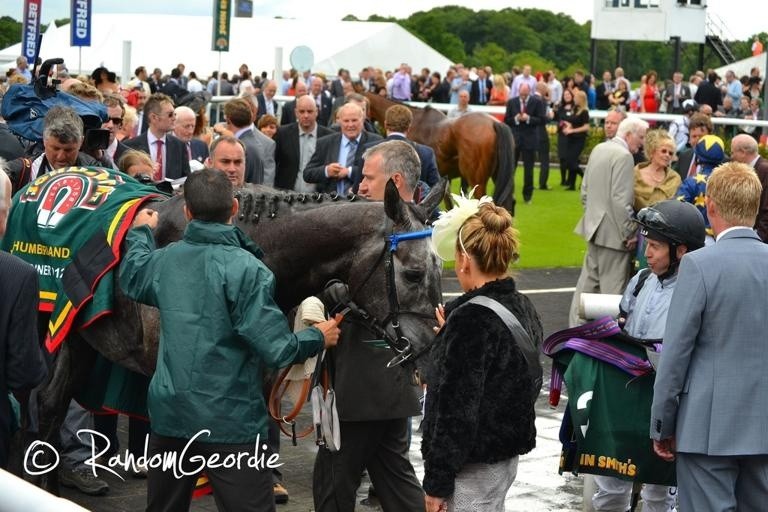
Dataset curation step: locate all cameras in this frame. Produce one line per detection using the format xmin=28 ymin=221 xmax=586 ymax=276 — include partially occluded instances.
xmin=133 ymin=172 xmax=174 ymax=195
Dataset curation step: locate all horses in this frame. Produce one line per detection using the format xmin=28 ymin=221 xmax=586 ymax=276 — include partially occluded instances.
xmin=1 ymin=165 xmax=448 ymax=503
xmin=360 ymin=92 xmax=516 ymax=214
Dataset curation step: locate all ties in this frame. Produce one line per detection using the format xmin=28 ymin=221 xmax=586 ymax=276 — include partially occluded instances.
xmin=522 ymin=100 xmax=526 ymax=114
xmin=344 ymin=140 xmax=358 ymax=195
xmin=153 ymin=139 xmax=163 ymax=182
xmin=482 ymin=80 xmax=484 ymax=103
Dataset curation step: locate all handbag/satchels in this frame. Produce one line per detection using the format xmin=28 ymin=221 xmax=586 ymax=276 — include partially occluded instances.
xmin=542 ymin=315 xmax=677 ymax=486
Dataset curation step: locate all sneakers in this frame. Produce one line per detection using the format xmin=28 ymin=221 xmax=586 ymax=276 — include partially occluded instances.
xmin=193 ymin=472 xmax=212 ymax=499
xmin=63 ymin=469 xmax=109 ymax=497
xmin=131 ymin=461 xmax=147 ymax=476
xmin=360 ymin=486 xmax=380 ymax=505
xmin=274 ymin=483 xmax=288 ymax=503
xmin=522 ymin=180 xmax=576 ymax=202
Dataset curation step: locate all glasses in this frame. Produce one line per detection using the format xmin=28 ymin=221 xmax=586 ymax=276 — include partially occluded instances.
xmin=656 ymin=149 xmax=673 ymax=156
xmin=153 ymin=113 xmax=176 ymax=118
xmin=707 ymin=113 xmax=712 ymax=116
xmin=102 ymin=113 xmax=122 ymax=124
xmin=635 ymin=207 xmax=671 ymax=231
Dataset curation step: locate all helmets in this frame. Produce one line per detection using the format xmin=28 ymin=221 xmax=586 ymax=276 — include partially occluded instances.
xmin=696 ymin=135 xmax=724 ymax=165
xmin=637 ymin=201 xmax=705 ymax=249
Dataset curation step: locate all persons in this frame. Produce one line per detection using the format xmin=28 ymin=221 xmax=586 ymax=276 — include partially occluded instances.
xmin=1 ymin=55 xmax=767 ymax=240
xmin=312 ymin=141 xmax=442 ymax=511
xmin=751 ymin=36 xmax=763 ymax=57
xmin=22 ymin=106 xmax=110 ymax=496
xmin=0 ymin=165 xmax=48 ymax=474
xmin=419 ymin=205 xmax=545 ymax=511
xmin=569 ymin=119 xmax=650 ymax=340
xmin=591 ymin=202 xmax=705 ymax=512
xmin=650 ymin=164 xmax=767 ymax=510
xmin=95 ymin=150 xmax=155 ymax=482
xmin=119 ymin=173 xmax=341 ymax=510
xmin=202 ymin=135 xmax=290 ymax=503
